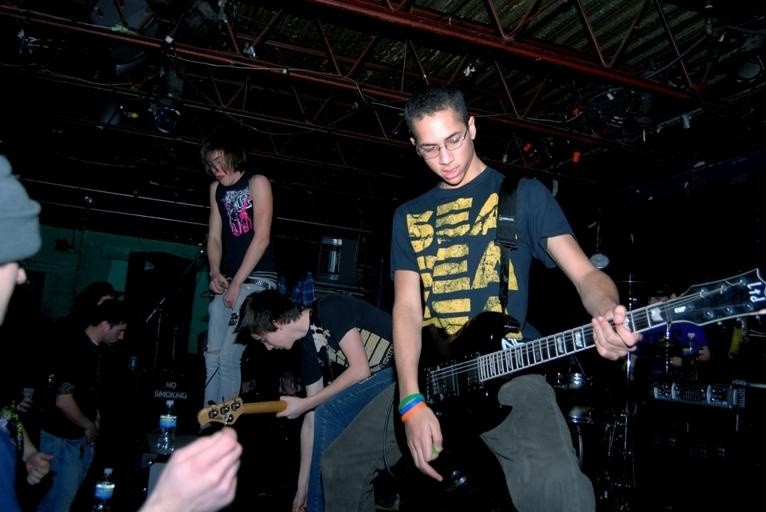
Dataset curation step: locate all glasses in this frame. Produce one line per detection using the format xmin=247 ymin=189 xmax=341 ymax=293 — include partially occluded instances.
xmin=415 ymin=130 xmax=468 ymax=160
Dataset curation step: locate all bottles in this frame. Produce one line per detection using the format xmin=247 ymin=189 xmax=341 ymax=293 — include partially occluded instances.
xmin=156 ymin=400 xmax=179 ymax=458
xmin=92 ymin=469 xmax=115 ymax=512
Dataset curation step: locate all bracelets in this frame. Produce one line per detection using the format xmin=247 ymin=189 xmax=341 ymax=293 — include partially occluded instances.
xmin=398 ymin=393 xmax=427 ymax=422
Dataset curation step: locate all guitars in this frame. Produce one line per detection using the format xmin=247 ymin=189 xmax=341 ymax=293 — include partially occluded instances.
xmin=197 ymin=394 xmax=288 ymax=428
xmin=393 ymin=268 xmax=761 ymax=463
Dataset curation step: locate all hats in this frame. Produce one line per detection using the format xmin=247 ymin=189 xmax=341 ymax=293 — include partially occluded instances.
xmin=0 ymin=155 xmax=41 ymax=264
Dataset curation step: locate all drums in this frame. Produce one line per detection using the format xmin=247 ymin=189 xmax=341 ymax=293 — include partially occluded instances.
xmin=549 ymin=367 xmax=598 ymax=424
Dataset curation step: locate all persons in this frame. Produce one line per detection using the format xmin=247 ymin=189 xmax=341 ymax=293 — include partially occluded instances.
xmin=622 ymin=281 xmax=712 ymax=381
xmin=197 ymin=135 xmax=279 ymax=436
xmin=233 ymin=287 xmax=393 ymax=512
xmin=0 ymin=153 xmax=244 ymax=511
xmin=320 ymin=87 xmax=643 ymax=512
